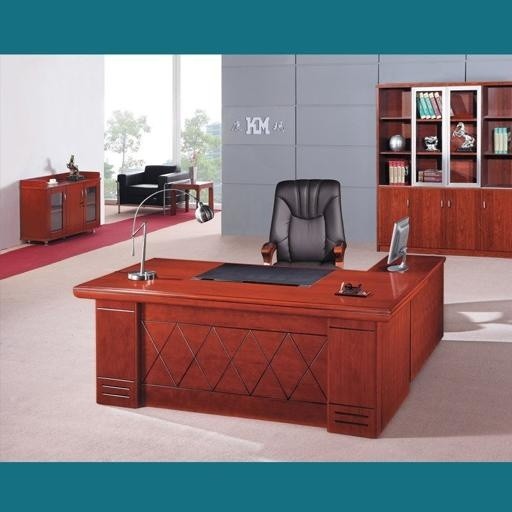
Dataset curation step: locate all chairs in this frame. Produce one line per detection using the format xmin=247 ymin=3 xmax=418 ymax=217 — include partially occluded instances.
xmin=261 ymin=179 xmax=346 ymax=268
xmin=117 ymin=165 xmax=190 ymax=216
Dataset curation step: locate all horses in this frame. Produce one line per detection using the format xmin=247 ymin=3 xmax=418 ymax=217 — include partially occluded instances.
xmin=452 ymin=121 xmax=475 ymax=149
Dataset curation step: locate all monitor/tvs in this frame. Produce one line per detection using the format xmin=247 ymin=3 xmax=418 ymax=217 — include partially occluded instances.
xmin=386 ymin=216 xmax=411 ymax=273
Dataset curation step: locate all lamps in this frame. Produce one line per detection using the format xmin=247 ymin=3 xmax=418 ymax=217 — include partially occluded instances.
xmin=127 ymin=189 xmax=214 ymax=281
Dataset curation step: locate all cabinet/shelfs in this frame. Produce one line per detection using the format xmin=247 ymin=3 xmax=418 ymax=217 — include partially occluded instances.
xmin=376 ymin=82 xmax=512 ymax=258
xmin=20 ymin=178 xmax=100 ymax=246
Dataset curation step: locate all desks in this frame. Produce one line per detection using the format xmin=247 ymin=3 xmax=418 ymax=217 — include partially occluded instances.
xmin=171 ymin=181 xmax=213 ymax=216
xmin=73 ymin=255 xmax=445 ymax=439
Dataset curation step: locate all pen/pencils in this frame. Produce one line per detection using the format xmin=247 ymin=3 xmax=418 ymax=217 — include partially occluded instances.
xmin=356 ymin=283 xmax=362 ymax=293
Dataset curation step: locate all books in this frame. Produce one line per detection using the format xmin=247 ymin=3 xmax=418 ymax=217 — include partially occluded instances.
xmin=389 ymin=158 xmax=409 ymax=186
xmin=415 ymin=92 xmax=456 ymax=120
xmin=493 ymin=127 xmax=511 ymax=154
xmin=417 ymin=168 xmax=443 ymax=183
